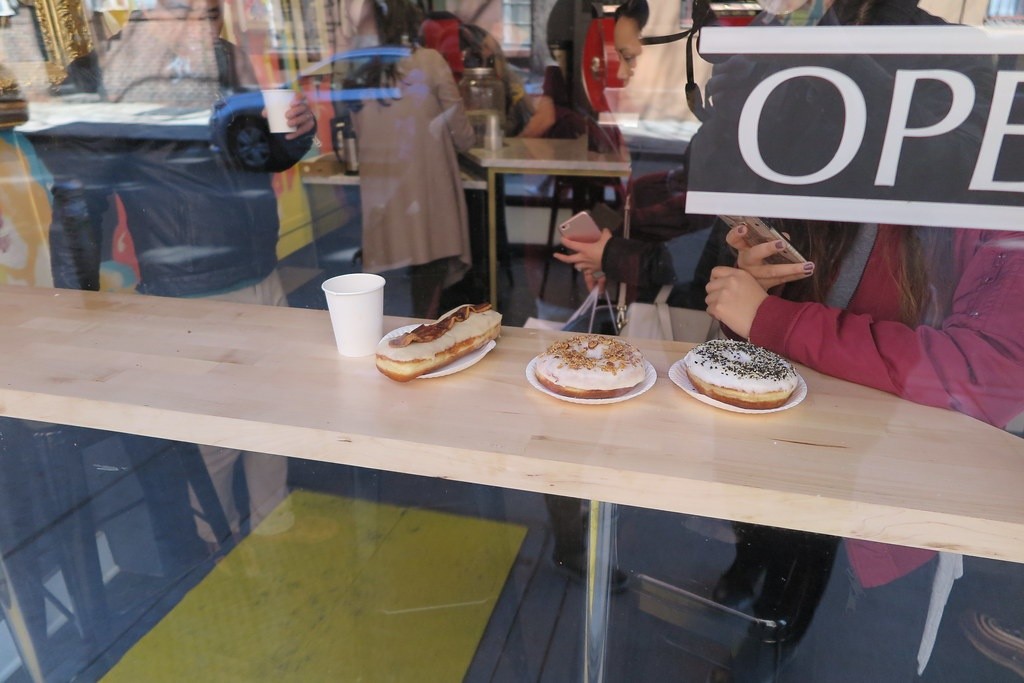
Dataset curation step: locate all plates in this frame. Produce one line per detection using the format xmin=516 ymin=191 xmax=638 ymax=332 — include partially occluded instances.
xmin=525 ymin=352 xmax=657 ymax=404
xmin=379 ymin=324 xmax=497 ymax=379
xmin=668 ymin=359 xmax=807 ymax=414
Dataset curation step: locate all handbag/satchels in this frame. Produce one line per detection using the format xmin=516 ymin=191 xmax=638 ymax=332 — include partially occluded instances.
xmin=522 ymin=284 xmax=618 ymax=336
xmin=619 ymin=283 xmax=730 ymax=342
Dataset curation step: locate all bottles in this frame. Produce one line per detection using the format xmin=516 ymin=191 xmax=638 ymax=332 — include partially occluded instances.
xmin=457 ymin=67 xmax=506 ymax=147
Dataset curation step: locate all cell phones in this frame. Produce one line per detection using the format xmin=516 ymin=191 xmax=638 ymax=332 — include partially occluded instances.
xmin=558 ymin=211 xmax=601 ymax=244
xmin=718 ymin=214 xmax=807 ymax=265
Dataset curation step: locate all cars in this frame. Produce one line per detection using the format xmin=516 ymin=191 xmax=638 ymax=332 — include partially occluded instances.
xmin=211 ymin=44 xmax=548 ymax=173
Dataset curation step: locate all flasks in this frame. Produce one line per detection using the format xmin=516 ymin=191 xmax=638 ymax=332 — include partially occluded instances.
xmin=331 ymin=117 xmax=358 ymax=175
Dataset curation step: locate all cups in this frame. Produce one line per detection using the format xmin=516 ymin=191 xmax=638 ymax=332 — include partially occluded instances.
xmin=260 ymin=89 xmax=298 ymax=133
xmin=483 ymin=113 xmax=503 ymax=150
xmin=321 ymin=273 xmax=386 ymax=359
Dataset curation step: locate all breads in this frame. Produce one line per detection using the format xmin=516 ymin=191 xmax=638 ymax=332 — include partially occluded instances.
xmin=682 ymin=339 xmax=798 ymax=411
xmin=374 ymin=303 xmax=503 ymax=383
xmin=534 ymin=334 xmax=646 ymax=398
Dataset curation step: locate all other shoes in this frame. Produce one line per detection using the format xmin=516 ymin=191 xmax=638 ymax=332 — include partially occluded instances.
xmin=555 ymin=556 xmax=629 ymax=591
xmin=582 ymin=506 xmax=620 ymax=528
xmin=713 ymin=560 xmax=758 ymax=605
xmin=729 ymin=627 xmax=799 ymax=683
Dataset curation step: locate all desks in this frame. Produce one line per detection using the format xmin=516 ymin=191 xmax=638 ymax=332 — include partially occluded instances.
xmin=470 ymin=137 xmax=632 ymax=313
xmin=0 ymin=286 xmax=1023 ymax=683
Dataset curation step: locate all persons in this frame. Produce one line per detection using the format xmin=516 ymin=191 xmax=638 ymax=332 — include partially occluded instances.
xmin=349 ymin=2 xmax=620 ymax=317
xmin=555 ymin=0 xmax=1024 ymax=641
xmin=0 ymin=1 xmax=318 ymax=567
